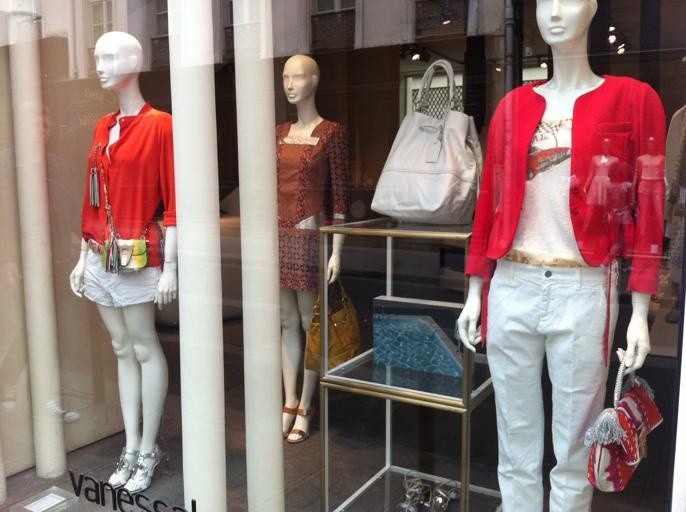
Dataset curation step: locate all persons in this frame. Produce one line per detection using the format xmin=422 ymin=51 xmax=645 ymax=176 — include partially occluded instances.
xmin=581 ymin=138 xmax=618 ymax=231
xmin=665 ymin=106 xmax=685 ymax=323
xmin=69 ymin=31 xmax=178 ymax=494
xmin=277 ymin=55 xmax=347 ymax=443
xmin=630 ymin=136 xmax=667 ymax=236
xmin=456 ymin=0 xmax=667 ymax=512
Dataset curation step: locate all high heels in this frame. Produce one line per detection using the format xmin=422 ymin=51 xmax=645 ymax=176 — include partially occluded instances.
xmin=288 ymin=402 xmax=316 ymax=444
xmin=283 ymin=403 xmax=298 ymax=442
xmin=392 ymin=468 xmax=425 ymax=511
xmin=120 ymin=444 xmax=162 ymax=495
xmin=106 ymin=444 xmax=139 ymax=490
xmin=421 ymin=479 xmax=464 ymax=511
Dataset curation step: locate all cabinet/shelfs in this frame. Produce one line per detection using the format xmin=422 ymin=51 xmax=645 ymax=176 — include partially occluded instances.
xmin=318 ymin=217 xmax=502 ymax=512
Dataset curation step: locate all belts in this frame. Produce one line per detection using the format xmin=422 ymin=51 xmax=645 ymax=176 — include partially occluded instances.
xmin=85 ymin=239 xmax=103 ymax=254
xmin=503 ymin=249 xmax=602 ymax=267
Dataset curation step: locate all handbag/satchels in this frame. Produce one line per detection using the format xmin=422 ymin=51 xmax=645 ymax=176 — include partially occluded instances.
xmin=369 ymin=55 xmax=488 ymax=226
xmin=101 ymin=237 xmax=150 ymax=274
xmin=585 ymin=355 xmax=665 ymax=495
xmin=305 ymin=273 xmax=365 ymax=376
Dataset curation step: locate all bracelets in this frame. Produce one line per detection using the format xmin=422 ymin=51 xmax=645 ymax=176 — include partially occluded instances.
xmin=166 ymin=260 xmax=174 ymax=263
